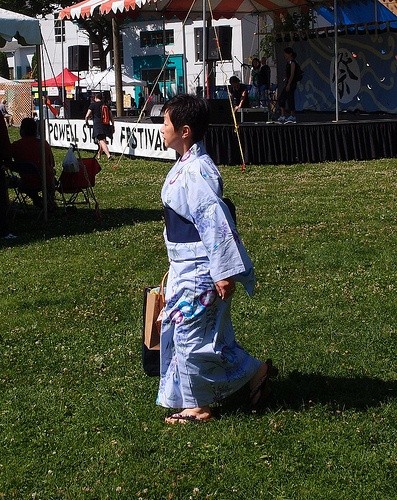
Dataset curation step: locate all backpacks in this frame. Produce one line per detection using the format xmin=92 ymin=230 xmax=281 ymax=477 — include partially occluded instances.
xmin=93 ymin=102 xmax=109 ymax=126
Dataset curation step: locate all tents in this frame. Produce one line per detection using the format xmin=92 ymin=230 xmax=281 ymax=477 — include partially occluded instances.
xmin=0 ymin=76 xmax=31 ymax=127
xmin=58 ymin=0 xmax=397 ymax=123
xmin=74 ymin=64 xmax=148 ymax=118
xmin=0 ymin=8 xmax=49 ymax=228
xmin=31 ymin=68 xmax=85 ymax=118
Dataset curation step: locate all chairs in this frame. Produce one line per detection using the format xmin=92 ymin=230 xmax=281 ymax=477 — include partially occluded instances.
xmin=5 ymin=162 xmax=67 ymax=229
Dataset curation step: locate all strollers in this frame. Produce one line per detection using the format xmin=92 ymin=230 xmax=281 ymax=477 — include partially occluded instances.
xmin=57 ymin=142 xmax=102 ymax=219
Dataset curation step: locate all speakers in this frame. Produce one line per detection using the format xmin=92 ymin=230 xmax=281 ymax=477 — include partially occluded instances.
xmin=199 ymin=25 xmax=233 ymax=62
xmin=68 ymin=45 xmax=89 ymax=71
xmin=64 ymin=99 xmax=83 ymax=119
xmin=149 ymin=104 xmax=164 ymax=123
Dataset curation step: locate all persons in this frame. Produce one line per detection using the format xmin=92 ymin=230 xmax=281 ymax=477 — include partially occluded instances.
xmin=1 ymin=99 xmax=11 ymax=127
xmin=248 ymin=58 xmax=262 ymax=108
xmin=0 ymin=108 xmax=18 ymax=241
xmin=128 ymin=92 xmax=146 ymax=117
xmin=255 ymin=56 xmax=271 ymax=97
xmin=85 ymin=93 xmax=115 ymax=162
xmin=12 ymin=118 xmax=59 ymax=214
xmin=156 ymin=94 xmax=280 ymax=425
xmin=228 ymin=76 xmax=251 ymax=121
xmin=276 ymin=47 xmax=298 ymax=123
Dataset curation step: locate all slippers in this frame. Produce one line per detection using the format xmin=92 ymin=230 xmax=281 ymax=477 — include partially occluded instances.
xmin=249 ymin=359 xmax=279 ymax=412
xmin=165 ymin=413 xmax=217 ymax=424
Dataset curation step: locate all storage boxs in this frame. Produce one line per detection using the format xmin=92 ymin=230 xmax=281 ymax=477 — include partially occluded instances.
xmin=236 ymin=106 xmax=270 ymax=125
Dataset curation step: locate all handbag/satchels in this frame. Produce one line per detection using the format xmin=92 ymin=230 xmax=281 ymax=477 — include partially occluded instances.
xmin=142 ymin=271 xmax=169 ymax=377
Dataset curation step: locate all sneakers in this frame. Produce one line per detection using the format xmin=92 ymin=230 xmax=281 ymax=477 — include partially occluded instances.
xmin=284 ymin=116 xmax=297 ymax=124
xmin=278 ymin=116 xmax=287 ymax=121
